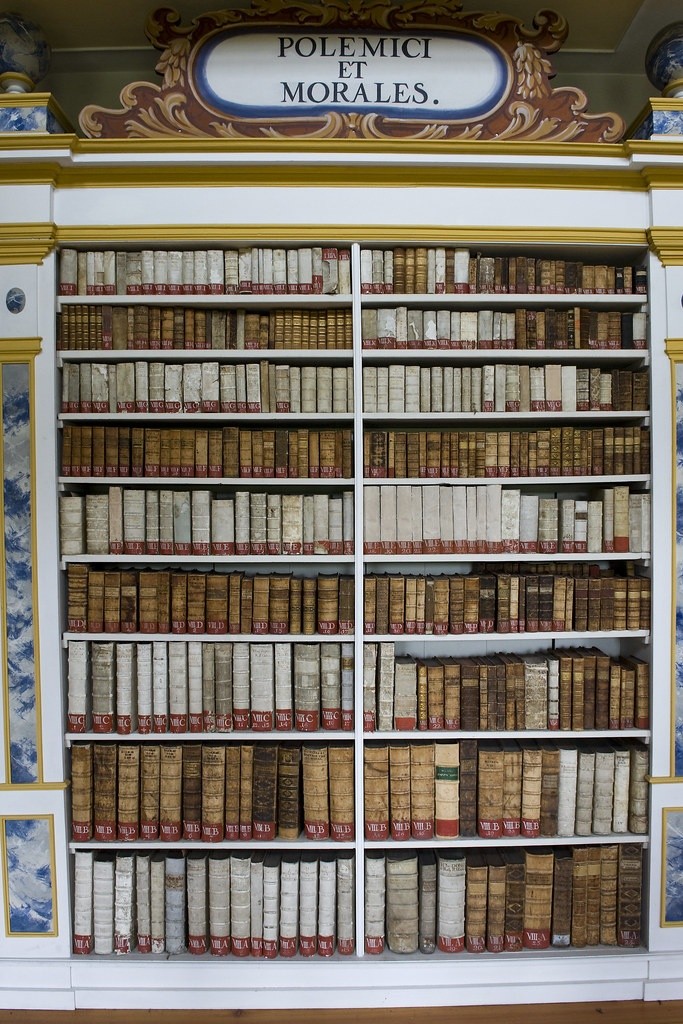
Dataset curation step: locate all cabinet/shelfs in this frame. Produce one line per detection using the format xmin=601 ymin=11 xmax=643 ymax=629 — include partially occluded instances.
xmin=0 ymin=133 xmax=683 ymax=1012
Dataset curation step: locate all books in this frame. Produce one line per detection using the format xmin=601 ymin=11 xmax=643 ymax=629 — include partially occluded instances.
xmin=58 ymin=485 xmax=355 ymax=556
xmin=362 ymin=426 xmax=650 ymax=477
xmin=71 ymin=743 xmax=355 ymax=844
xmin=61 ymin=305 xmax=352 ymax=351
xmin=61 ymin=424 xmax=354 ymax=479
xmin=363 ymin=560 xmax=651 ymax=636
xmin=364 ymin=485 xmax=651 ymax=554
xmin=61 ymin=360 xmax=354 ymax=413
xmin=362 ymin=304 xmax=648 ymax=349
xmin=364 ymin=642 xmax=651 ymax=731
xmin=67 ymin=562 xmax=355 ymax=636
xmin=68 ymin=642 xmax=354 ymax=734
xmin=363 ymin=738 xmax=651 ymax=841
xmin=363 ymin=363 xmax=649 ymax=413
xmin=73 ymin=849 xmax=355 ymax=958
xmin=365 ymin=843 xmax=644 ymax=955
xmin=361 ymin=245 xmax=649 ymax=295
xmin=58 ymin=246 xmax=351 ymax=296
